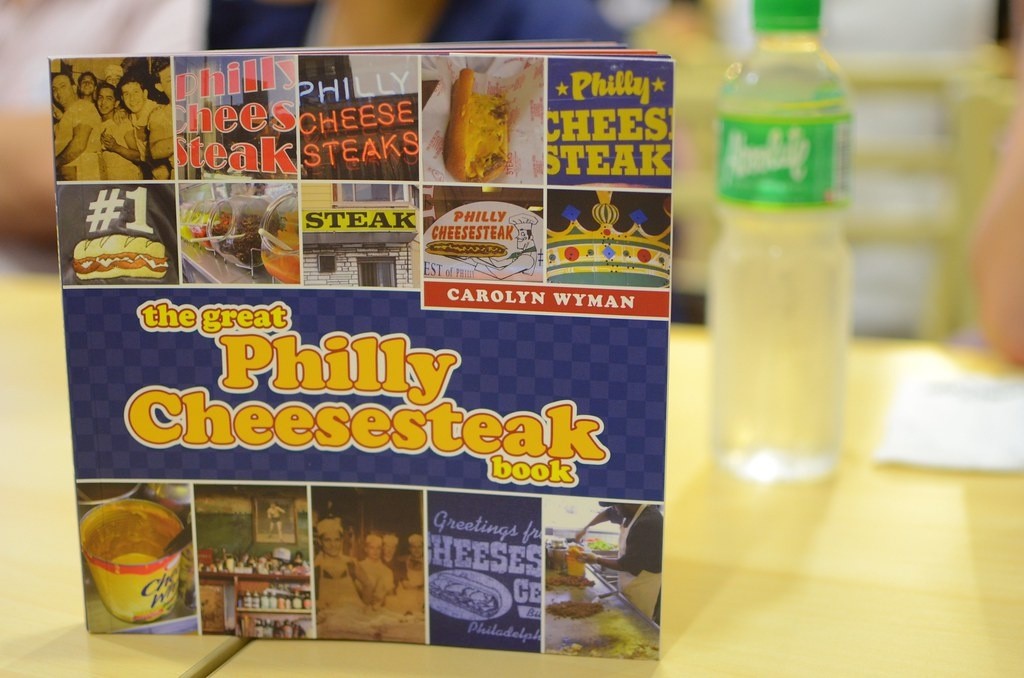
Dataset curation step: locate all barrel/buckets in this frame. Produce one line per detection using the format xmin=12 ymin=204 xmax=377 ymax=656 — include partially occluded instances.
xmin=546 ymin=545 xmax=568 ymax=571
xmin=81 ymin=499 xmax=184 ymax=626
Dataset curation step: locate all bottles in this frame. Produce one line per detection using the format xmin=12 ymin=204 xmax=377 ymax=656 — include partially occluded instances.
xmin=198 ymin=550 xmax=314 ymax=638
xmin=707 ymin=0 xmax=858 ymax=483
xmin=564 ymin=543 xmax=585 ymax=576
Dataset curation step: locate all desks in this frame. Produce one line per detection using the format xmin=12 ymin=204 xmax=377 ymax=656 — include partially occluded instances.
xmin=0 ymin=243 xmax=1023 ymax=677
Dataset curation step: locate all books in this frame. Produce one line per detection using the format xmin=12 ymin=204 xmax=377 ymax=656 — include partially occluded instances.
xmin=46 ymin=38 xmax=673 ymax=665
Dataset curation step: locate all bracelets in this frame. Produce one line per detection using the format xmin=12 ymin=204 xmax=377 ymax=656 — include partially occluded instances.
xmin=596 ymin=556 xmax=600 ymax=564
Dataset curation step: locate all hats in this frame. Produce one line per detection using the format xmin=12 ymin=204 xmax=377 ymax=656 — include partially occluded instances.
xmin=104 ymin=64 xmax=124 ymax=80
xmin=317 ymin=518 xmax=344 ymax=536
xmin=367 ymin=535 xmax=382 ymax=544
xmin=384 ymin=535 xmax=399 ymax=544
xmin=409 ymin=534 xmax=422 ymax=543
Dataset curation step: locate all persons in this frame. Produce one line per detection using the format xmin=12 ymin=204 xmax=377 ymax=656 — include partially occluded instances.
xmin=203 ymin=3 xmax=633 ymax=50
xmin=263 ymin=501 xmax=287 ymax=542
xmin=50 ymin=57 xmax=176 ymax=183
xmin=311 ymin=519 xmax=424 ymax=615
xmin=572 ymin=500 xmax=663 ymax=623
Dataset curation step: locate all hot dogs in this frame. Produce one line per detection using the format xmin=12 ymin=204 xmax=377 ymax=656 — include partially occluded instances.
xmin=440 ymin=67 xmax=510 ymax=185
